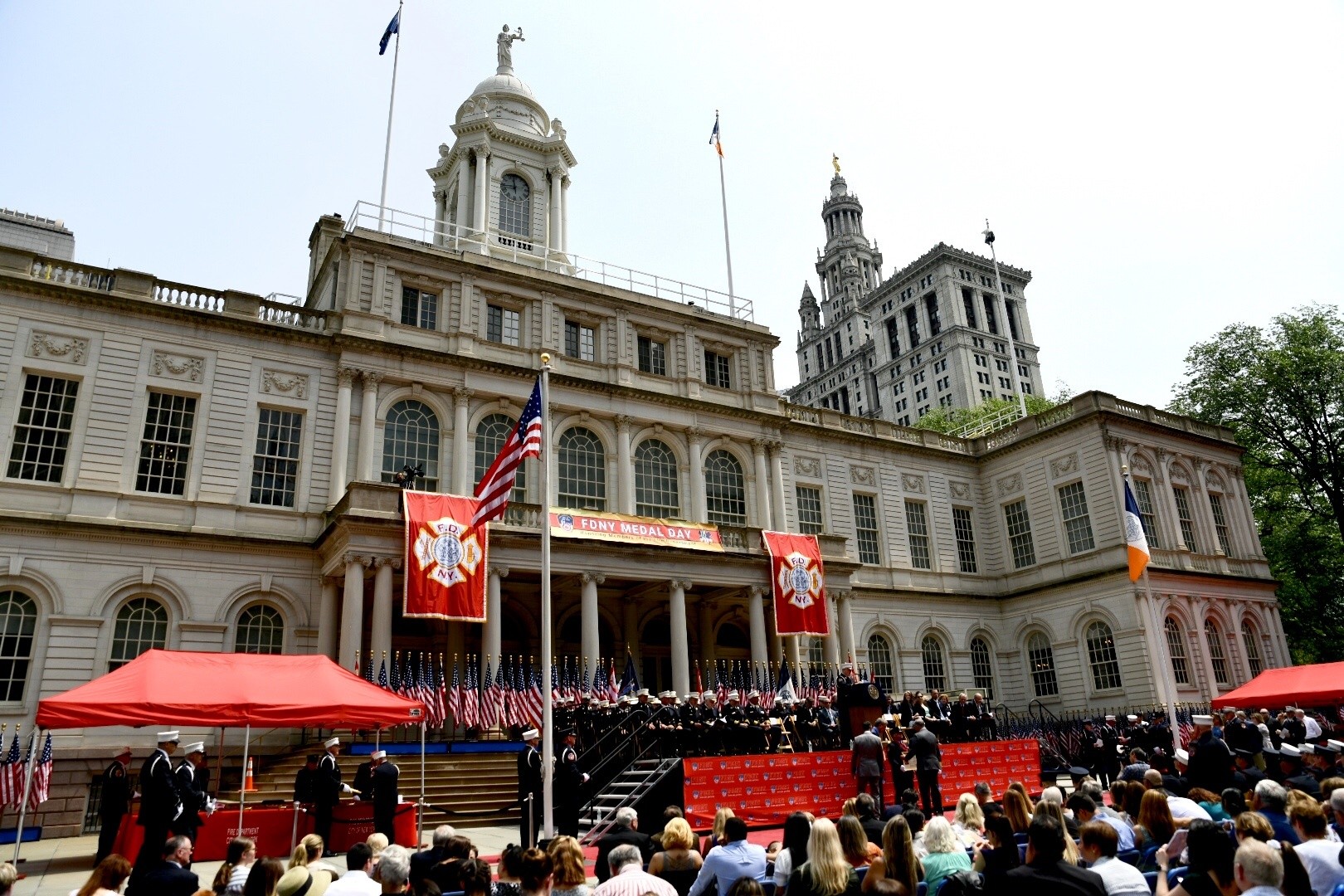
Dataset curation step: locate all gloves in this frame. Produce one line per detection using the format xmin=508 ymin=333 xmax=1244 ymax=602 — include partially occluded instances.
xmin=582 ymin=773 xmax=590 ymax=782
xmin=132 ymin=792 xmax=141 ymax=798
xmin=204 ymin=795 xmax=215 ymax=815
xmin=353 ymin=795 xmax=360 ymax=800
xmin=173 ymin=804 xmax=184 ymax=821
xmin=341 ymin=782 xmax=352 ymax=794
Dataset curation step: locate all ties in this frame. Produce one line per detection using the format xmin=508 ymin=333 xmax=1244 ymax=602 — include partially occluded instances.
xmin=962 ymin=704 xmax=964 ymax=710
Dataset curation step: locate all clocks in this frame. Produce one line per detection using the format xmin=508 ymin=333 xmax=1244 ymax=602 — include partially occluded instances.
xmin=500 ymin=173 xmax=529 ymax=203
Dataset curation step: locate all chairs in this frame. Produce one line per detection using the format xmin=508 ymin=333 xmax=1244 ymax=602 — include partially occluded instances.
xmin=760 ymin=833 xmax=1190 ymax=896
xmin=686 ymin=713 xmax=939 ymax=759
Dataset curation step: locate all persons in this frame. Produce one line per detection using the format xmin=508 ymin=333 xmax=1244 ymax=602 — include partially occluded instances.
xmin=832 ymin=153 xmax=841 ymax=174
xmin=497 ymin=24 xmax=522 ymax=67
xmin=0 ymin=863 xmax=17 ymax=896
xmin=72 ymin=664 xmax=1344 ymax=896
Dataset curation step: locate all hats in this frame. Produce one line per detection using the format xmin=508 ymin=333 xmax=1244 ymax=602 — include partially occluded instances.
xmin=112 ymin=746 xmax=133 ymax=757
xmin=522 ymin=728 xmax=542 ymax=740
xmin=372 ymin=750 xmax=386 ymax=760
xmin=272 ymin=865 xmax=333 ymax=896
xmin=889 ymin=727 xmax=903 ymax=737
xmin=323 ymin=737 xmax=342 ymax=749
xmin=157 ymin=731 xmax=181 ymax=742
xmin=371 ymin=751 xmax=378 ymax=757
xmin=183 ymin=741 xmax=206 ymax=754
xmin=553 ymin=688 xmax=837 ymax=710
xmin=557 ymin=727 xmax=576 ymax=737
xmin=306 ymin=754 xmax=320 ymax=763
xmin=841 ymin=663 xmax=853 ymax=670
xmin=1069 ymin=706 xmax=1344 ymax=784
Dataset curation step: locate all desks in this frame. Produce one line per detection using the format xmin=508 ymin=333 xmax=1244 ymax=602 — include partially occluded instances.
xmin=114 ymin=802 xmax=418 ymax=869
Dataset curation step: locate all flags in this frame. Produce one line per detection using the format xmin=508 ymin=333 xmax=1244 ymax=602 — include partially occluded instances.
xmin=695 ymin=653 xmax=875 ymax=709
xmin=471 ymin=373 xmax=543 ymax=530
xmin=354 ymin=651 xmax=643 ymax=742
xmin=709 ymin=116 xmax=724 ymax=158
xmin=0 ymin=728 xmax=53 ymax=813
xmin=379 ymin=10 xmax=399 ymax=56
xmin=1123 ymin=473 xmax=1151 ymax=581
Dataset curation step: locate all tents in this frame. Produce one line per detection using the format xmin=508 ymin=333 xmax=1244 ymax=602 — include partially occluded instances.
xmin=12 ymin=649 xmax=428 ymax=868
xmin=1210 ymin=660 xmax=1344 ymax=714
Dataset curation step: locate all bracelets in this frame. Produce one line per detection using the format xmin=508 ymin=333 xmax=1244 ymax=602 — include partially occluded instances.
xmin=1158 ymin=865 xmax=1168 ymax=871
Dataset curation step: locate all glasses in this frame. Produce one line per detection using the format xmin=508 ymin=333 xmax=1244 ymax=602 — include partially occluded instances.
xmin=917 ymin=696 xmax=923 ymax=699
xmin=245 ymin=848 xmax=258 ymax=853
xmin=182 ymin=847 xmax=194 ymax=852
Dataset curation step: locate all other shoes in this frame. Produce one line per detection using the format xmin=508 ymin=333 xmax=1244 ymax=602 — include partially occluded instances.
xmin=933 ymin=809 xmax=943 ymax=816
